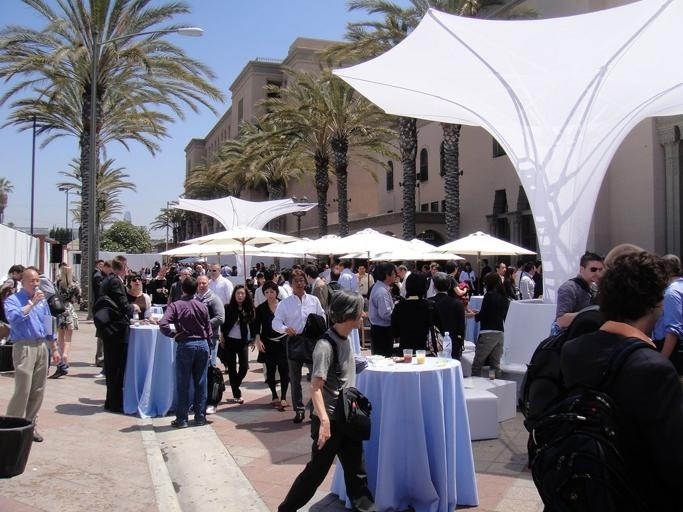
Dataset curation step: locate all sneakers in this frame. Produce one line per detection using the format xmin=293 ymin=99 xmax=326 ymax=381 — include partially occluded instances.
xmin=48 ymin=369 xmax=67 ymax=379
xmin=170 ymin=396 xmax=305 ymax=428
xmin=32 ymin=429 xmax=43 ymax=442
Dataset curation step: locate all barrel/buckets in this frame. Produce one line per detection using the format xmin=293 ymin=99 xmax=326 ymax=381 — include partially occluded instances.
xmin=0 ymin=414 xmax=36 ymax=480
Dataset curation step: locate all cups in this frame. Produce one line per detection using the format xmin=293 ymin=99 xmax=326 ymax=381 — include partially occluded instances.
xmin=437 ymin=352 xmax=447 ymax=367
xmin=403 ymin=349 xmax=413 ymax=363
xmin=416 ymin=350 xmax=427 ymax=365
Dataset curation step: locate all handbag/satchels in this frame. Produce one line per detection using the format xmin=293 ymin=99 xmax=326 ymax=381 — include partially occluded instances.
xmin=207 ymin=366 xmax=225 ymax=407
xmin=91 ymin=296 xmax=130 ymax=338
xmin=332 ymin=386 xmax=371 ymax=442
xmin=216 ymin=342 xmax=232 ymax=365
xmin=46 ymin=293 xmax=65 ymax=317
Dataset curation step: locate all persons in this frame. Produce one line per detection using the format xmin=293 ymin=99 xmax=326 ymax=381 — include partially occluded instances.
xmin=524 ymin=251 xmax=682 ymax=512
xmin=95 ymin=254 xmax=543 ymax=428
xmin=4 ymin=269 xmax=61 ymax=423
xmin=556 ymin=244 xmax=682 ymax=379
xmin=0 ymin=263 xmax=81 ymax=379
xmin=275 ymin=290 xmax=380 ymax=512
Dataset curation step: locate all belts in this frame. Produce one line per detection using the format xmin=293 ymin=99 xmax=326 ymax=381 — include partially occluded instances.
xmin=186 ymin=335 xmax=204 ymax=339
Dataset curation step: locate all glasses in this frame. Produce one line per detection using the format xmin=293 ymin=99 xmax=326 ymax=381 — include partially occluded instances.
xmin=590 ymin=266 xmax=603 ymax=273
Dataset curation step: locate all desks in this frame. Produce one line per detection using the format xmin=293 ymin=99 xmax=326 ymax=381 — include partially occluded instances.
xmin=330 ymin=357 xmax=479 ymax=512
xmin=464 ymin=295 xmax=484 ymax=346
xmin=123 ymin=324 xmax=194 ymax=418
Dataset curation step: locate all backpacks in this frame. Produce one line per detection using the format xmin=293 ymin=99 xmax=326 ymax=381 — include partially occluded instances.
xmin=514 ymin=328 xmax=658 ymax=512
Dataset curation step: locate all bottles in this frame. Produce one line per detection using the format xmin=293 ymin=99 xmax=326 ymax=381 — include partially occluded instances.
xmin=0 ymin=336 xmax=7 ymax=347
xmin=34 ymin=286 xmax=47 ymax=306
xmin=442 ymin=332 xmax=453 ymax=363
xmin=133 ymin=312 xmax=140 ymax=327
